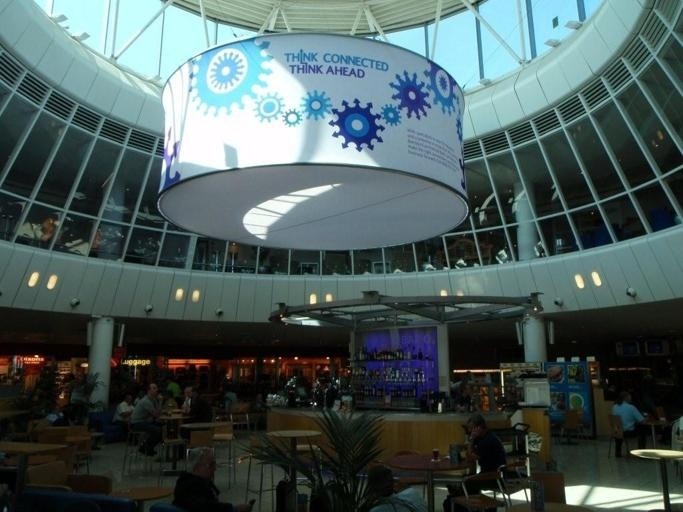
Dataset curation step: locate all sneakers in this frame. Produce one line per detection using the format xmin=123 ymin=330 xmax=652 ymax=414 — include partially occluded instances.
xmin=138 ymin=444 xmax=158 ymax=457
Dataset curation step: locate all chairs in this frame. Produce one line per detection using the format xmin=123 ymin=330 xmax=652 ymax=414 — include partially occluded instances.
xmin=560 ymin=409 xmax=577 ymax=445
xmin=606 ymin=414 xmax=629 ymax=458
xmin=0 ymin=407 xmax=530 ymax=512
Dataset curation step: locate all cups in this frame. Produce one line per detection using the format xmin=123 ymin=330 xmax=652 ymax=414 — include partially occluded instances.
xmin=384 ymin=392 xmax=391 ymax=407
xmin=340 ymin=395 xmax=353 ymax=412
xmin=432 ymin=449 xmax=439 ymax=460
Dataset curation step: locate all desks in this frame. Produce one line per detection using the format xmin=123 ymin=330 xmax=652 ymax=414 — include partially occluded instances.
xmin=642 ymin=419 xmax=666 ymax=448
xmin=629 ymin=447 xmax=683 ymax=512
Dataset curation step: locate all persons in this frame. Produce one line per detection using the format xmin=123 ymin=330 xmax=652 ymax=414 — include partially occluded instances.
xmin=462 ymin=415 xmax=507 ymax=494
xmin=173 ymin=447 xmax=255 ymax=512
xmin=612 ymin=388 xmax=647 ymax=459
xmin=112 ymin=382 xmax=213 ymax=456
xmin=318 ymin=377 xmax=341 ymax=412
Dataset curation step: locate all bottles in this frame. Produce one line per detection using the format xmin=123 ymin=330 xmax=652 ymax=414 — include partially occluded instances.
xmin=419 ymin=389 xmax=476 ymax=415
xmin=352 ymin=342 xmax=426 ymax=398
xmin=166 ymin=399 xmax=174 ymax=417
